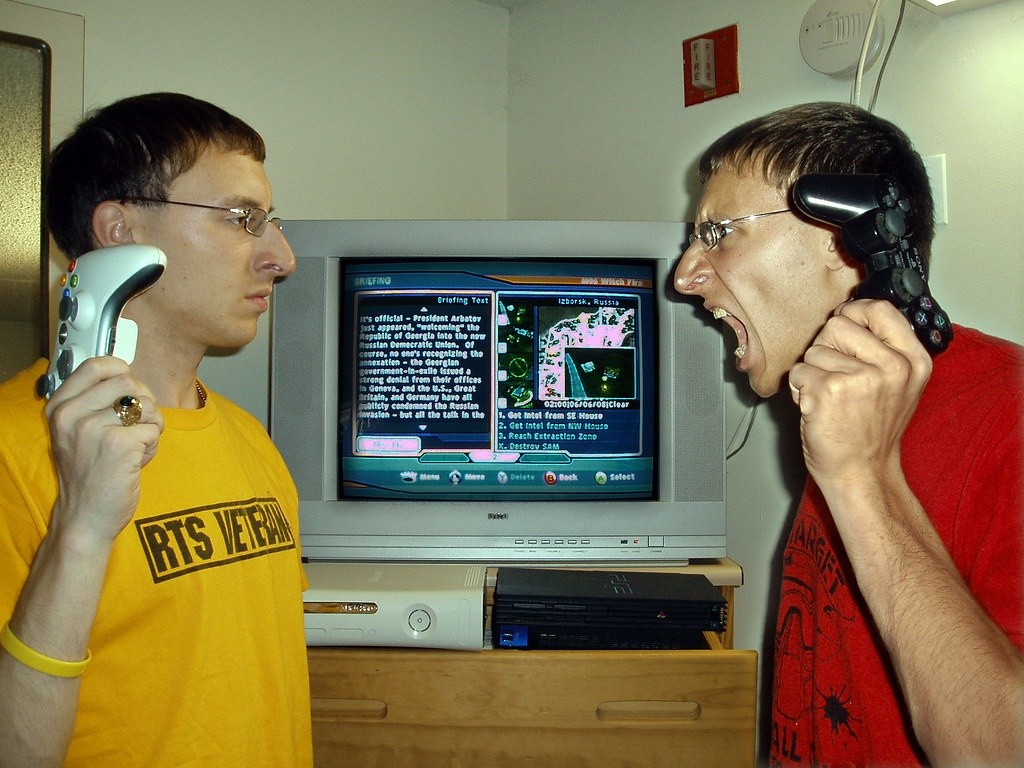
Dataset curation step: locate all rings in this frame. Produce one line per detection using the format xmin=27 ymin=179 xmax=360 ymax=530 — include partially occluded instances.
xmin=114 ymin=395 xmax=142 ymax=426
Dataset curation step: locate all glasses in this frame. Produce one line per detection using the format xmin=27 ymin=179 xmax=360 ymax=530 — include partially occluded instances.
xmin=89 ymin=194 xmax=283 ymax=238
xmin=688 ymin=208 xmax=792 ymax=246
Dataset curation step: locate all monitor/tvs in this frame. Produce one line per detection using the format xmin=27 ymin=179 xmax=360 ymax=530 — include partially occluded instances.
xmin=271 ymin=220 xmax=727 ymax=568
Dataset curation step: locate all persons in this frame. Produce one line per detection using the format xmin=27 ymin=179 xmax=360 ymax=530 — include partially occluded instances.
xmin=0 ymin=94 xmax=314 ymax=768
xmin=675 ymin=101 xmax=1024 ymax=768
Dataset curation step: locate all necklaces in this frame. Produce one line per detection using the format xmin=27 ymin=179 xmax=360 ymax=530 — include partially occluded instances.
xmin=196 ymin=382 xmax=207 ymax=405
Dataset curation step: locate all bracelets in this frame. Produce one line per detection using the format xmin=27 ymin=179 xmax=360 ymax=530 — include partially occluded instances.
xmin=0 ymin=622 xmax=92 ymax=678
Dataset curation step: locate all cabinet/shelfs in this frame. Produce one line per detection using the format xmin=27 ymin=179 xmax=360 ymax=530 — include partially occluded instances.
xmin=304 ymin=557 xmax=757 ymax=768
